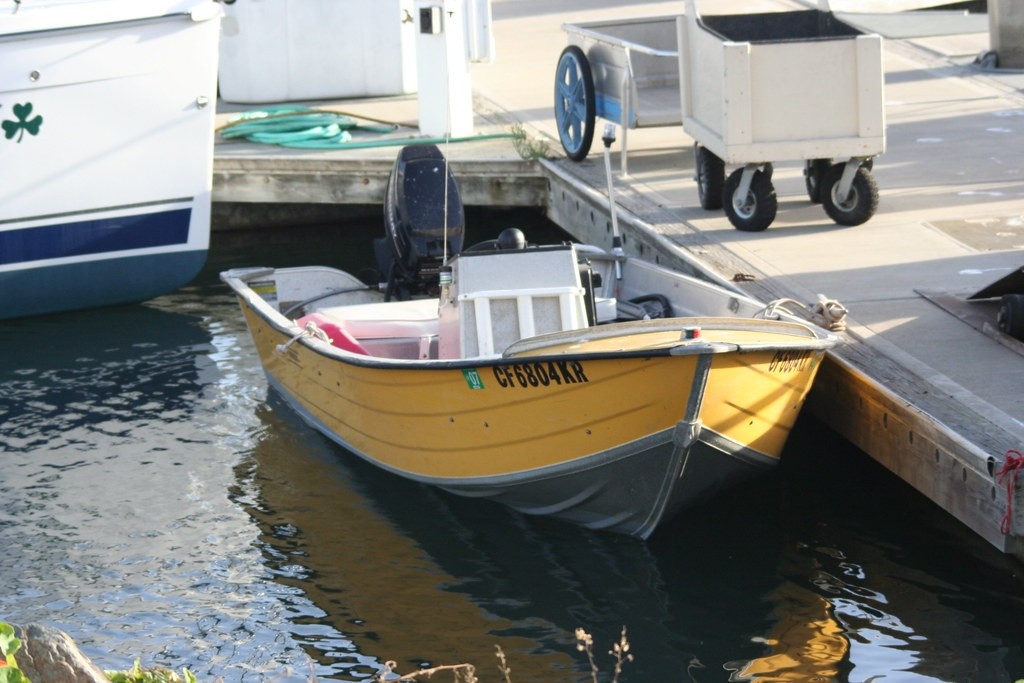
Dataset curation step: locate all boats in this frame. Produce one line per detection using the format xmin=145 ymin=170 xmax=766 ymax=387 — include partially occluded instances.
xmin=1 ymin=0 xmax=223 ymax=323
xmin=217 ymin=139 xmax=839 ymax=543
